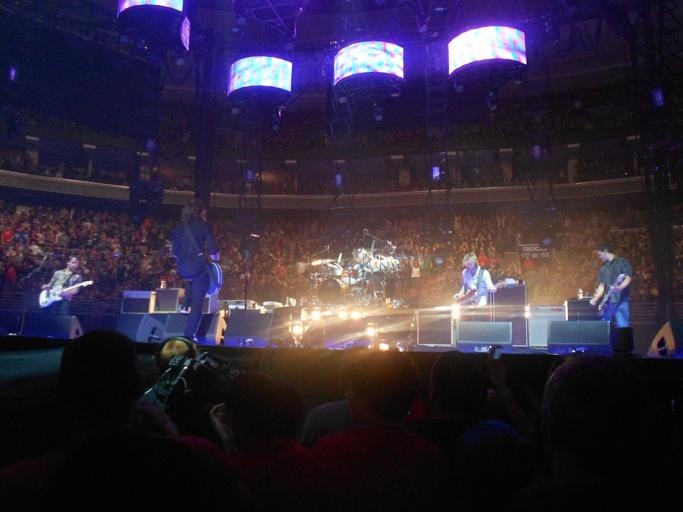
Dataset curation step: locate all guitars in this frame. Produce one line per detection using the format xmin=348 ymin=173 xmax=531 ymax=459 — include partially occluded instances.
xmin=39 ymin=280 xmax=94 ymax=308
xmin=455 ymin=280 xmax=507 ymax=306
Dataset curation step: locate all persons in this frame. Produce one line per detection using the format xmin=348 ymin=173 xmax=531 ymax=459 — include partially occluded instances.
xmin=37 ymin=254 xmax=83 ymax=338
xmin=587 ymin=242 xmax=635 ymax=356
xmin=451 ymin=252 xmax=498 ymax=322
xmin=0 ymin=328 xmax=683 ymax=512
xmin=165 ymin=198 xmax=224 ymax=345
xmin=0 ymin=96 xmax=683 ymax=195
xmin=0 ymin=202 xmax=682 ymax=314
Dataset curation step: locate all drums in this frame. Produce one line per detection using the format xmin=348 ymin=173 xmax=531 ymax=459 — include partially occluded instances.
xmin=317 ymin=278 xmax=353 ymax=306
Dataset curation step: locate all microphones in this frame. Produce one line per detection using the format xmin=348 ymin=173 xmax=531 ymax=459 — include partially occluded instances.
xmin=41 ymin=256 xmax=50 ymax=268
